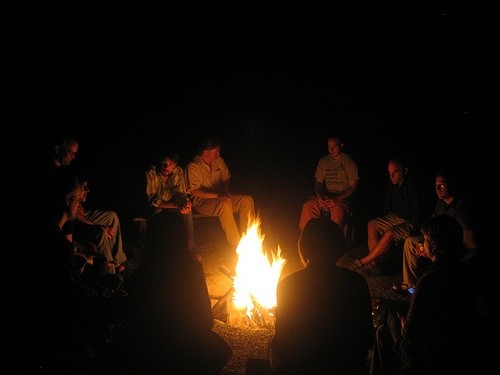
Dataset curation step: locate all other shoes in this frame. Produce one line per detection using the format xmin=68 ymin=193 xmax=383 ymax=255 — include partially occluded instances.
xmin=392 ymin=282 xmax=413 ymax=290
xmin=336 ymin=259 xmax=363 ymax=272
xmin=359 ymin=261 xmax=376 ymax=271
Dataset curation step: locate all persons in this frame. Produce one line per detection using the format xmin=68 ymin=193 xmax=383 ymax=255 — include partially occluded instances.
xmin=185 ymin=133 xmax=260 ymax=252
xmin=363 ymin=214 xmax=485 ymax=372
xmin=47 ymin=175 xmax=120 ymax=282
xmin=397 ymin=161 xmax=470 ymax=285
xmin=295 ymin=135 xmax=360 ymax=239
xmin=116 ymin=208 xmax=229 ymax=372
xmin=37 ymin=135 xmax=83 ymax=209
xmin=353 ymin=154 xmax=421 ymax=273
xmin=445 ymin=198 xmax=498 ymax=304
xmin=268 ymin=217 xmax=372 ymax=372
xmin=130 ymin=150 xmax=196 ymax=263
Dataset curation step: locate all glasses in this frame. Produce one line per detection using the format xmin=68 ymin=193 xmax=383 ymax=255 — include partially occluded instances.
xmin=434 ymin=183 xmax=447 ymax=189
xmin=63 ymin=147 xmax=76 ymax=156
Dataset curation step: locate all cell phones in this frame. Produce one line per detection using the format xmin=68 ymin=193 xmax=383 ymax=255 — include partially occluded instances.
xmin=407 ymin=287 xmax=414 ymax=295
